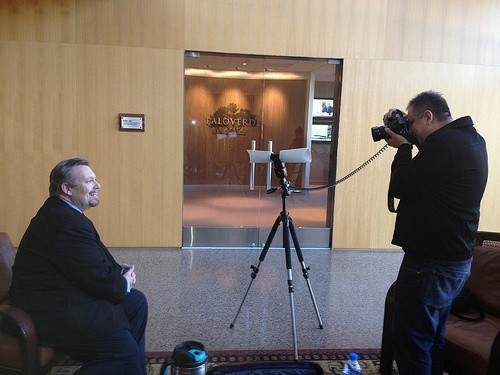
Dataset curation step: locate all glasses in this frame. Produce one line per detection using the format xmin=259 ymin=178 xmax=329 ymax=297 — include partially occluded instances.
xmin=409 ymin=115 xmax=424 ymax=126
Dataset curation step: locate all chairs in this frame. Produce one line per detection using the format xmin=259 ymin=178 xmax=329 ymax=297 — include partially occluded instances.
xmin=380 ymin=231 xmax=500 ymax=375
xmin=0 ymin=232 xmax=62 ymax=375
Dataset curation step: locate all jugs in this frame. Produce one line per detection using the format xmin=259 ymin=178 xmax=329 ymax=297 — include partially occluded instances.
xmin=160 ymin=341 xmax=209 ymax=375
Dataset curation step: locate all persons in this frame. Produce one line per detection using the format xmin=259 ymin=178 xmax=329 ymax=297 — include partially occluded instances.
xmin=382 ymin=90 xmax=489 ymax=375
xmin=8 ymin=158 xmax=149 ymax=375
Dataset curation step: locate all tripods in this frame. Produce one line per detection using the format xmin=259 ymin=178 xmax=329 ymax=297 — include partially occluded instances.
xmin=229 ymin=178 xmax=323 ymax=362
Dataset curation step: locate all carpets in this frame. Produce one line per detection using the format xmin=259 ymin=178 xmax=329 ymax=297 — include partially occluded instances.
xmin=47 ymin=348 xmax=384 ymax=375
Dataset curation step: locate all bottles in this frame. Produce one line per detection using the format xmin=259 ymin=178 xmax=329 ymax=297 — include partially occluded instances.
xmin=343 ymin=353 xmax=362 ymax=375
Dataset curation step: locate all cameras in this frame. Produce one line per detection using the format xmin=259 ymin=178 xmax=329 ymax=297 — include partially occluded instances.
xmin=371 ymin=109 xmax=413 ymax=144
